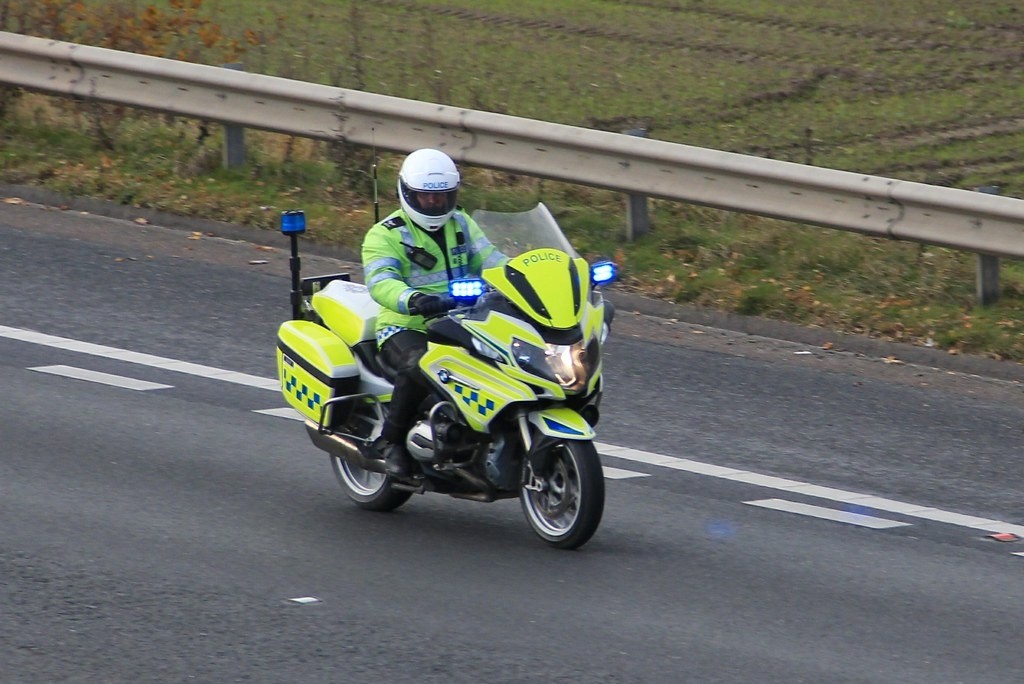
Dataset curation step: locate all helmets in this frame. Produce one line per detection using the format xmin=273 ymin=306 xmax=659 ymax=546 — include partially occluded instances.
xmin=397 ymin=148 xmax=461 ymax=231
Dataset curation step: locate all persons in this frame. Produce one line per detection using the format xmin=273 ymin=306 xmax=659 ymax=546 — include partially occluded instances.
xmin=361 ymin=148 xmax=515 ymax=478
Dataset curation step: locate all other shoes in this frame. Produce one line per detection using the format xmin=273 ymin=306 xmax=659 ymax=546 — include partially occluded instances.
xmin=375 ymin=438 xmax=423 ymax=476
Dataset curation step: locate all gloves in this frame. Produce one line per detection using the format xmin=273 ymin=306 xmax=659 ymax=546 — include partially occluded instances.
xmin=409 ymin=292 xmax=448 ymax=319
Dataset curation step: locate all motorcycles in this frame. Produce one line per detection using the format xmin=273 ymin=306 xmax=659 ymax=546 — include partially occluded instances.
xmin=275 ymin=200 xmax=622 ymax=551
